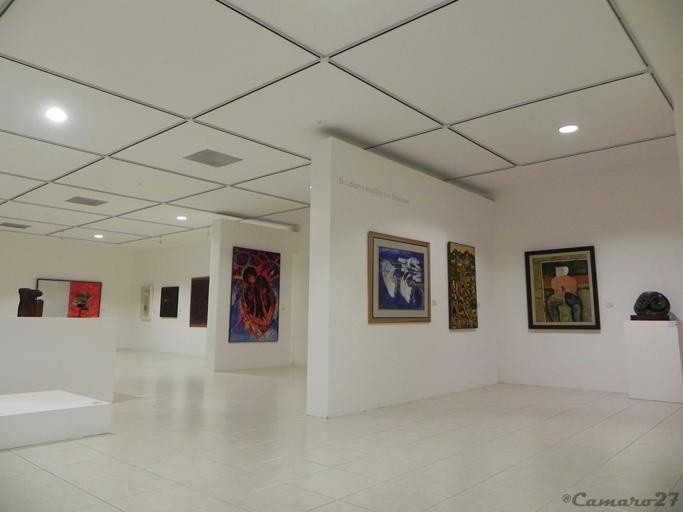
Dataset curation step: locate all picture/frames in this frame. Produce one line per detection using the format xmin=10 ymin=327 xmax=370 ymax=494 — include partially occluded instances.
xmin=140 ymin=276 xmax=209 ymax=327
xmin=227 ymin=246 xmax=281 ymax=344
xmin=523 ymin=246 xmax=602 ymax=330
xmin=34 ymin=278 xmax=102 ymax=318
xmin=367 ymin=230 xmax=478 ymax=330
